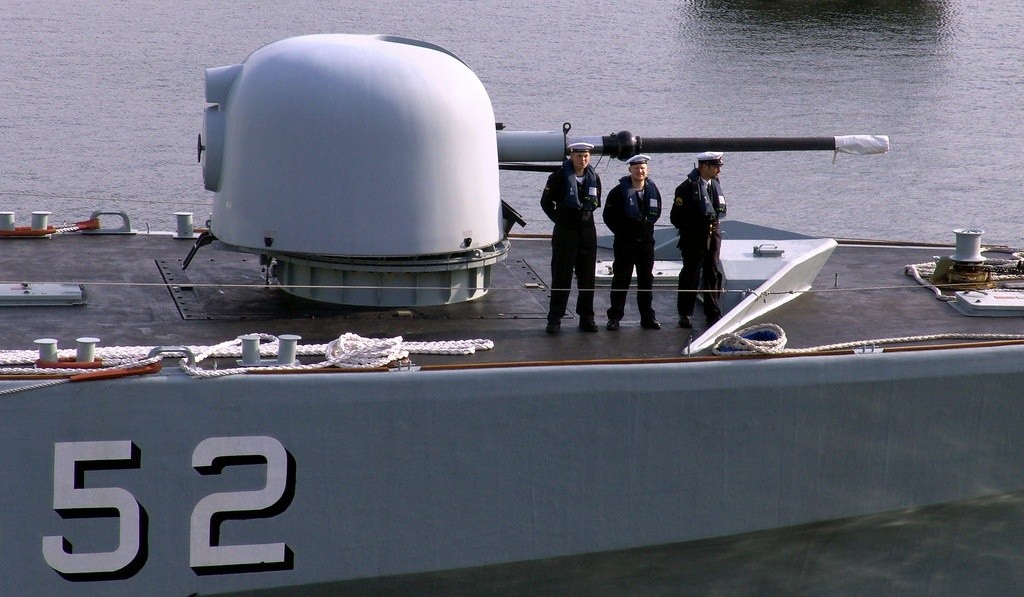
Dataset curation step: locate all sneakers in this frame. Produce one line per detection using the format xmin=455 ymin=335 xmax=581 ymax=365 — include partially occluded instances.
xmin=579 ymin=318 xmax=598 ymax=332
xmin=546 ymin=316 xmax=560 ymax=332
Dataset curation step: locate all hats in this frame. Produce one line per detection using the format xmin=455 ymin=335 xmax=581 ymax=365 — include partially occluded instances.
xmin=696 ymin=151 xmax=724 ymax=167
xmin=625 ymin=155 xmax=651 ymax=165
xmin=568 ymin=143 xmax=594 ymax=153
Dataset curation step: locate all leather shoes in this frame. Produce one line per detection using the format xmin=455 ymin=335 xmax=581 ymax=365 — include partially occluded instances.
xmin=641 ymin=319 xmax=661 ymax=329
xmin=607 ymin=318 xmax=619 ymax=330
xmin=679 ymin=317 xmax=692 ymax=329
xmin=706 ymin=317 xmax=721 ymax=324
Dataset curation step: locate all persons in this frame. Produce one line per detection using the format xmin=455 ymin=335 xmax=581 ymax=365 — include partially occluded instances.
xmin=604 ymin=154 xmax=664 ymax=331
xmin=668 ymin=152 xmax=729 ymax=329
xmin=543 ymin=142 xmax=603 ymax=337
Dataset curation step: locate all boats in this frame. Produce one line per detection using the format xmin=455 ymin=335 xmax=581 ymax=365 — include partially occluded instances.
xmin=0 ymin=33 xmax=1024 ymax=597
